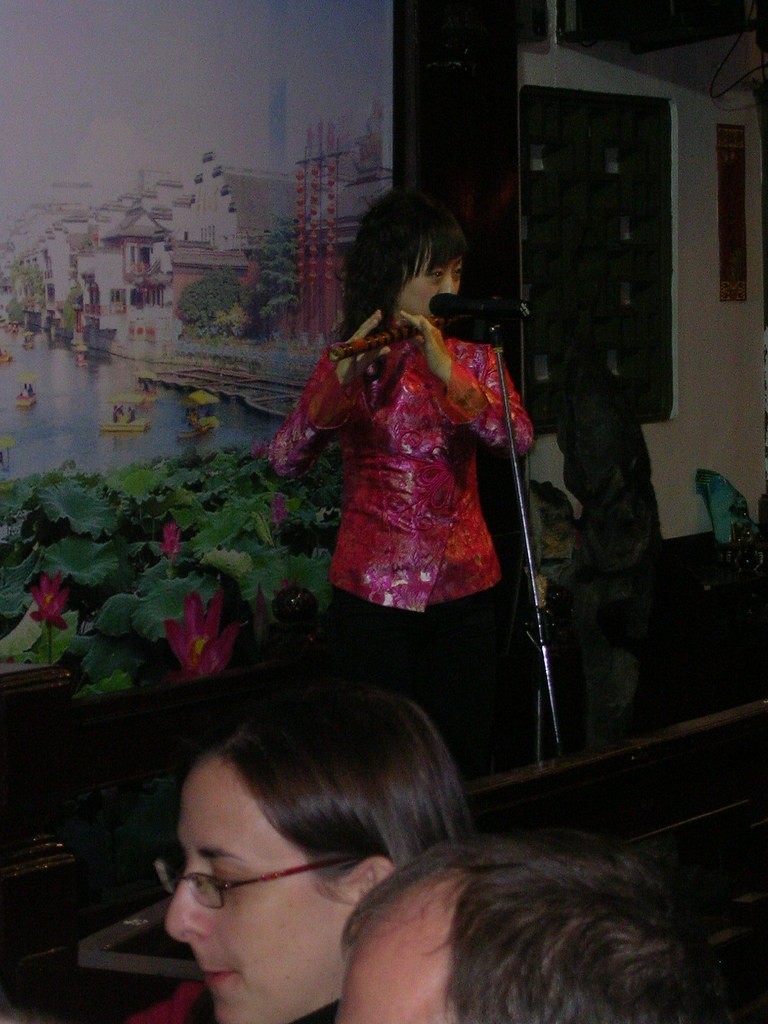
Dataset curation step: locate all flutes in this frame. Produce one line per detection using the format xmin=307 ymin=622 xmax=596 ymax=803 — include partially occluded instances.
xmin=328 ymin=296 xmax=504 ymax=363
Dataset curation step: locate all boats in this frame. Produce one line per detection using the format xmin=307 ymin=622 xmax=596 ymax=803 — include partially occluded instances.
xmin=99 ymin=391 xmax=157 ymax=435
xmin=15 ymin=371 xmax=40 ymax=407
xmin=177 ymin=389 xmax=222 ymax=439
xmin=0 ymin=436 xmax=16 ymax=479
xmin=72 ymin=344 xmax=91 ymax=369
xmin=110 ymin=372 xmax=162 ymax=406
xmin=0 ymin=318 xmax=37 ymax=364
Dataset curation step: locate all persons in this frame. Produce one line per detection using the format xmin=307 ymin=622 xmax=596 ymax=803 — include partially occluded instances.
xmin=337 ymin=829 xmax=740 ymax=1023
xmin=270 ymin=197 xmax=535 ymax=783
xmin=127 ymin=690 xmax=478 ymax=1024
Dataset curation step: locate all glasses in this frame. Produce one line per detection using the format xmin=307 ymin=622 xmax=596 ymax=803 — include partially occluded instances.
xmin=154 ymin=856 xmax=352 ymax=908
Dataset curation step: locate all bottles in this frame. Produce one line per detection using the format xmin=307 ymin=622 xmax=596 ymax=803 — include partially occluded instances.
xmin=735 ymin=524 xmax=757 ymax=574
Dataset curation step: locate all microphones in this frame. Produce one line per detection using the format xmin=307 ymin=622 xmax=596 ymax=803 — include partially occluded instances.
xmin=429 ymin=292 xmax=524 ymax=318
